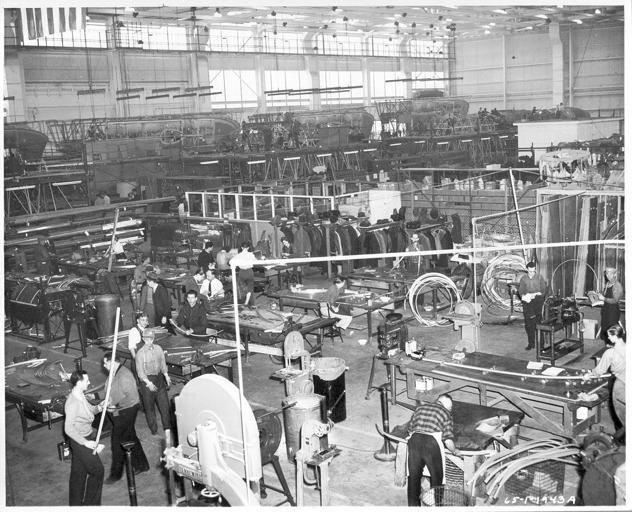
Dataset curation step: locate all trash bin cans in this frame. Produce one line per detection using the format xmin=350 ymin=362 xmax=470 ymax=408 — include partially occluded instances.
xmin=95 ymin=294 xmax=122 ymax=337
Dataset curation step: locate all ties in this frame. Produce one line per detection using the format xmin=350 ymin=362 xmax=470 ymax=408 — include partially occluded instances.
xmin=207 ymin=281 xmax=211 ymax=297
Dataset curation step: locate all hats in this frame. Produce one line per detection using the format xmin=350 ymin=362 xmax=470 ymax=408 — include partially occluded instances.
xmin=410 ymin=233 xmax=420 ymax=241
xmin=141 ymin=328 xmax=157 ymax=339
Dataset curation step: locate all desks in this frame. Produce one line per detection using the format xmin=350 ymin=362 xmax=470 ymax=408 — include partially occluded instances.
xmin=6 ymin=329 xmax=247 ymax=461
xmin=207 ymin=267 xmax=463 ymax=367
xmin=388 ymin=398 xmax=527 ymax=498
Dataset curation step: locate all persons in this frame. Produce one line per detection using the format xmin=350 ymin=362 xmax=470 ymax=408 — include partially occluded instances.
xmin=583 ymin=324 xmax=625 ymax=425
xmin=596 ymin=264 xmax=623 ymax=346
xmin=102 ymin=352 xmax=151 ymax=487
xmin=84 ymin=102 xmax=567 ymax=149
xmin=127 ymin=312 xmax=150 ymax=414
xmin=407 ymin=394 xmax=461 ymax=506
xmin=63 ymin=368 xmax=113 ymax=506
xmin=5 ymin=186 xmax=428 ymax=344
xmin=134 ymin=327 xmax=172 ymax=436
xmin=518 ymin=262 xmax=548 ymax=351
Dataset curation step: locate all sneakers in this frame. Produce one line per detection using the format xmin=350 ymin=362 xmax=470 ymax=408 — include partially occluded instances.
xmin=104 ymin=464 xmax=150 ymax=485
xmin=525 ymin=342 xmax=535 ymax=351
